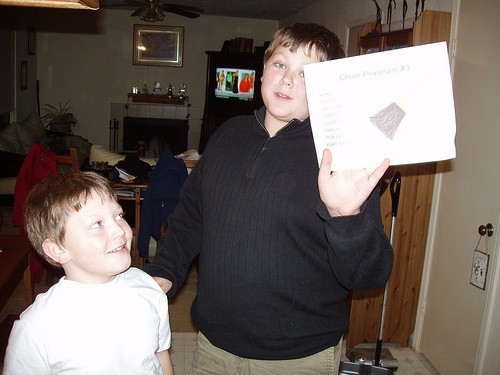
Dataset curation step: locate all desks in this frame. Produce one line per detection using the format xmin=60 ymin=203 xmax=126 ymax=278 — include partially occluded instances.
xmin=0 ymin=233 xmax=35 ymax=307
xmin=111 ymin=178 xmax=148 ymax=268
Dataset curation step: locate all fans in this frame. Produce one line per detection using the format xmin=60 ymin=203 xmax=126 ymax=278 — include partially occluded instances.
xmin=124 ymin=0 xmax=205 ymax=19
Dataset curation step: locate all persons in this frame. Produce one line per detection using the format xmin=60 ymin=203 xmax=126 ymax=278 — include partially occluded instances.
xmin=148 ymin=24 xmax=393 ymax=375
xmin=217 ymin=70 xmax=254 ymax=95
xmin=2 ymin=172 xmax=173 ymax=375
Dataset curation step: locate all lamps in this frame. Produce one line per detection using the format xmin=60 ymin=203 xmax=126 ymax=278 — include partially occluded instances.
xmin=139 ymin=6 xmax=166 ymax=22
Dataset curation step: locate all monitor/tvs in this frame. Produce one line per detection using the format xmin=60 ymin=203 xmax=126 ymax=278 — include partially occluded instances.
xmin=214 ymin=65 xmax=260 ymax=105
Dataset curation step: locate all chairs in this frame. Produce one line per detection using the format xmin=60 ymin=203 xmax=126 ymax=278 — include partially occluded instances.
xmin=13 ymin=144 xmax=80 ymax=288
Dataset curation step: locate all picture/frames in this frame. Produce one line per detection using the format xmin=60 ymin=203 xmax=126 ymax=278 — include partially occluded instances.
xmin=132 ymin=23 xmax=185 ymax=68
xmin=20 ymin=60 xmax=28 ymax=90
xmin=27 ymin=26 xmax=35 ymax=55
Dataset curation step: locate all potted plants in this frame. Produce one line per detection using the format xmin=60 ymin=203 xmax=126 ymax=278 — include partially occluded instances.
xmin=40 ymin=97 xmax=78 ymax=133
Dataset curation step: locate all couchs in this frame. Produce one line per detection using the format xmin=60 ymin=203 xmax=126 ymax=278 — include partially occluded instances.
xmin=0 ymin=123 xmax=92 ymax=171
xmin=88 ymin=144 xmax=200 ymax=177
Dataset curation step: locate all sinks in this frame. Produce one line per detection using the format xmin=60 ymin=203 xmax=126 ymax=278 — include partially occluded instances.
xmin=125 ymin=95 xmax=190 ymax=121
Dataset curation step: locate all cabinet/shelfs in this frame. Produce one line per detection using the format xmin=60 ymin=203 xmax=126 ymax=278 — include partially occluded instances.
xmin=200 ymin=50 xmax=265 ymax=155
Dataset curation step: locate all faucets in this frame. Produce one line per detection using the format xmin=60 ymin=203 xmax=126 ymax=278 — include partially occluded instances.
xmin=151 ymin=80 xmax=162 ymax=95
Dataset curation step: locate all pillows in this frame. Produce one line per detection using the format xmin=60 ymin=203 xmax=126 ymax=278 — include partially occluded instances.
xmin=17 ymin=113 xmax=45 ymax=151
